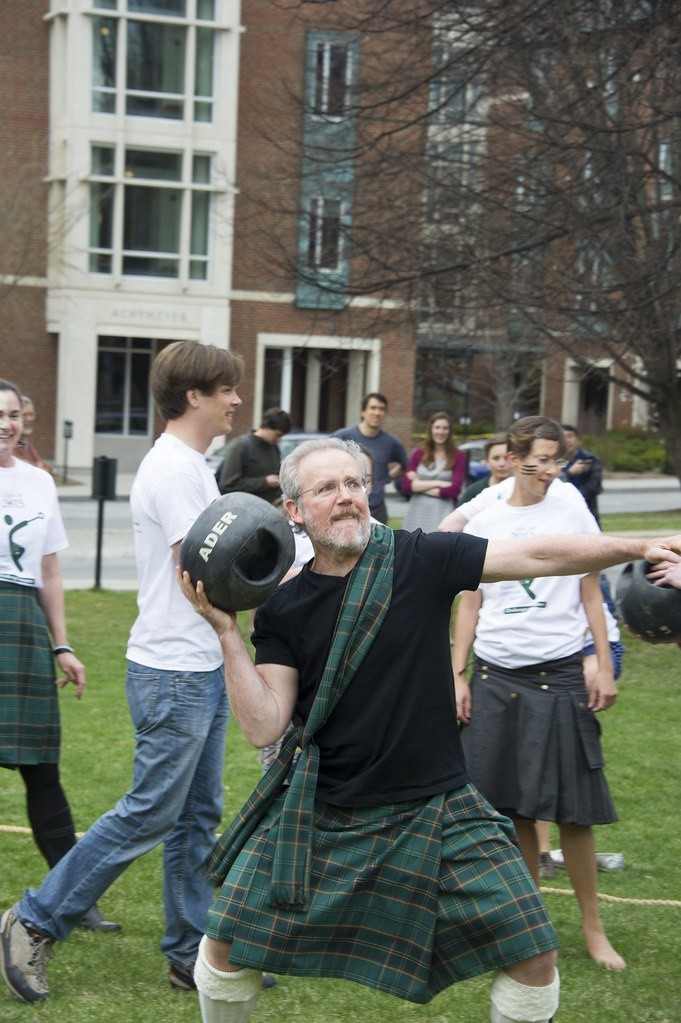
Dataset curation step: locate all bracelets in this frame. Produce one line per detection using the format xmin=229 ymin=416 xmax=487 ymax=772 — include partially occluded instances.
xmin=52 ymin=645 xmax=74 ymax=654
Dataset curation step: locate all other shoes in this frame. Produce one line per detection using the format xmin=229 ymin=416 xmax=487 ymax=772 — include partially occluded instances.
xmin=536 ymin=850 xmax=555 ymax=879
xmin=77 ymin=903 xmax=122 ymax=933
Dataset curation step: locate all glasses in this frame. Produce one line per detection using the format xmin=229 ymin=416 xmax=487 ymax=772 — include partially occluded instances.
xmin=531 ymin=457 xmax=568 ymax=470
xmin=294 ymin=477 xmax=368 ymax=497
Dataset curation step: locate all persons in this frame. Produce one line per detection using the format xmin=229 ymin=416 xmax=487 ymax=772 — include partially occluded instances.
xmin=0 ymin=341 xmax=276 ymax=1002
xmin=219 ymin=408 xmax=292 ymax=503
xmin=277 ymin=393 xmax=408 ymax=587
xmin=176 ymin=437 xmax=681 ymax=1023
xmin=647 ymin=559 xmax=681 ymax=588
xmin=439 ymin=416 xmax=626 ymax=972
xmin=0 ymin=381 xmax=122 ymax=927
xmin=13 ymin=396 xmax=53 ymax=475
xmin=402 ymin=413 xmax=467 ymax=534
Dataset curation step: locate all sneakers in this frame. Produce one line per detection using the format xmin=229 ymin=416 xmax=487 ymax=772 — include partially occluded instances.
xmin=0 ymin=906 xmax=50 ymax=1004
xmin=168 ymin=961 xmax=276 ymax=991
